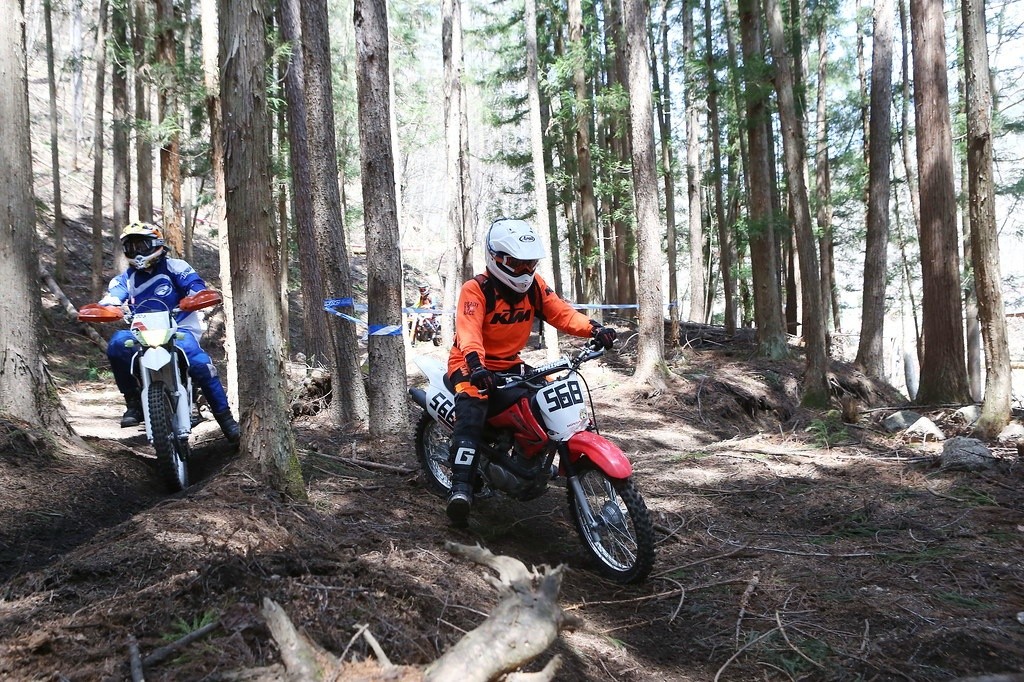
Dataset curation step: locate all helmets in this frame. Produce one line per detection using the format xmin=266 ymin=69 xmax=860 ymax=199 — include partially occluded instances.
xmin=119 ymin=220 xmax=166 ymax=270
xmin=418 ymin=282 xmax=430 ymax=297
xmin=484 ymin=217 xmax=546 ymax=294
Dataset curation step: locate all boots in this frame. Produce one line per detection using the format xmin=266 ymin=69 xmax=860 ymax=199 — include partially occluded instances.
xmin=542 ymin=450 xmax=561 ymax=479
xmin=210 ymin=406 xmax=241 ymax=443
xmin=446 ymin=441 xmax=480 ymax=529
xmin=120 ymin=387 xmax=143 ymax=427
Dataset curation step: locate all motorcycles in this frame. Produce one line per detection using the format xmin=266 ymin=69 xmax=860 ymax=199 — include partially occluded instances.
xmin=405 ymin=298 xmax=442 ymax=346
xmin=75 ymin=291 xmax=222 ymax=500
xmin=404 ymin=335 xmax=657 ymax=588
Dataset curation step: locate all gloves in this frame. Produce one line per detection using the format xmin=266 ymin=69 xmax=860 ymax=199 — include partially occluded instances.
xmin=590 ymin=326 xmax=617 ymax=351
xmin=469 ymin=365 xmax=498 ymax=395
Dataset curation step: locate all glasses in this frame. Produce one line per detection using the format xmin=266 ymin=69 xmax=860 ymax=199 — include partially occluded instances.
xmin=486 ymin=219 xmax=541 ymax=274
xmin=122 ymin=239 xmax=164 ymax=257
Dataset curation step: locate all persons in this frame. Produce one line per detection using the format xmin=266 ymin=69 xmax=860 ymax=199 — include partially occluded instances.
xmin=446 ymin=216 xmax=617 ymax=521
xmin=410 ymin=282 xmax=436 ymax=347
xmin=96 ymin=219 xmax=242 ymax=444
